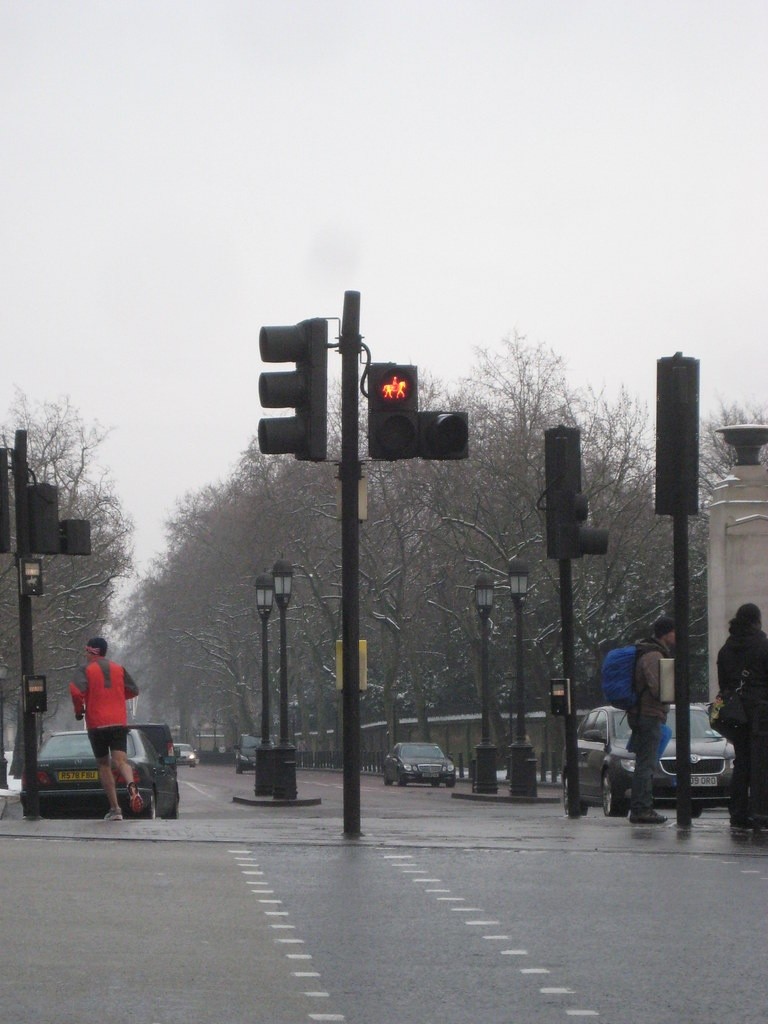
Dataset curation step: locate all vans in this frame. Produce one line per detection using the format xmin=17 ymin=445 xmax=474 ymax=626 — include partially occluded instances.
xmin=126 ymin=724 xmax=178 ymax=776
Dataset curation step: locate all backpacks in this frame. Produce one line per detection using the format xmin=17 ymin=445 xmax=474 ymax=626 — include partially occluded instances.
xmin=600 ymin=644 xmax=668 ymax=711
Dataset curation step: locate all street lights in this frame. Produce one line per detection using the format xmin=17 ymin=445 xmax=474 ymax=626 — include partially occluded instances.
xmin=197 ymin=723 xmax=202 ymax=751
xmin=474 ymin=571 xmax=499 ymax=795
xmin=507 ymin=553 xmax=535 ymax=796
xmin=270 ymin=552 xmax=299 ymax=801
xmin=0 ymin=656 xmax=9 ymax=790
xmin=254 ymin=568 xmax=275 ymax=795
xmin=212 ymin=718 xmax=217 ymax=747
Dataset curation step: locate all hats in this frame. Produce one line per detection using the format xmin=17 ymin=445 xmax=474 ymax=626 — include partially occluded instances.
xmin=86 ymin=638 xmax=107 ymax=656
xmin=654 ymin=616 xmax=675 ymax=634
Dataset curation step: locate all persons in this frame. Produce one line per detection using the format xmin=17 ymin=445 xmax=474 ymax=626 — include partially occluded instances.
xmin=716 ymin=603 xmax=768 ymax=828
xmin=627 ymin=617 xmax=675 ymax=823
xmin=69 ymin=638 xmax=145 ymax=821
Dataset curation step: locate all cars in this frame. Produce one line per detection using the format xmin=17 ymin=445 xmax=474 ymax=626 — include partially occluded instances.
xmin=562 ymin=706 xmax=737 ymax=818
xmin=233 ymin=736 xmax=263 ymax=774
xmin=19 ymin=730 xmax=179 ymax=820
xmin=383 ymin=743 xmax=456 ymax=787
xmin=174 ymin=743 xmax=197 ymax=767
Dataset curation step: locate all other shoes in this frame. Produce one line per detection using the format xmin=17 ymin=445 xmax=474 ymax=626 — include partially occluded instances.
xmin=630 ymin=808 xmax=667 ymax=823
xmin=730 ymin=816 xmax=768 ymax=829
xmin=104 ymin=808 xmax=122 ymax=820
xmin=127 ymin=782 xmax=143 ymax=812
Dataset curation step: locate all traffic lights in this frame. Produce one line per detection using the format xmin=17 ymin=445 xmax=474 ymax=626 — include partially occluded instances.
xmin=420 ymin=411 xmax=470 ymax=461
xmin=23 ymin=675 xmax=47 ymax=713
xmin=550 ymin=678 xmax=571 ymax=717
xmin=258 ymin=317 xmax=328 ymax=462
xmin=20 ymin=557 xmax=43 ymax=596
xmin=366 ymin=362 xmax=418 ymax=462
xmin=545 ymin=424 xmax=609 ymax=560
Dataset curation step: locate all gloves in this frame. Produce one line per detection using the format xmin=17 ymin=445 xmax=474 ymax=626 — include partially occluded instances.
xmin=76 ymin=706 xmax=85 ymax=720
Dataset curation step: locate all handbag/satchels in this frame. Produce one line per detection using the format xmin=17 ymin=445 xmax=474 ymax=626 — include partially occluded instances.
xmin=704 ymin=690 xmax=748 ymax=740
